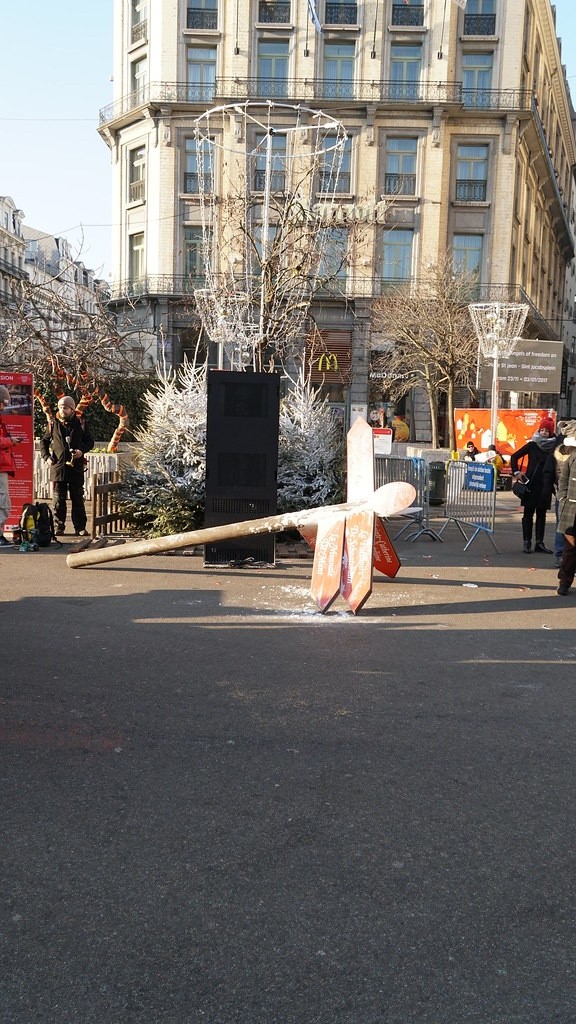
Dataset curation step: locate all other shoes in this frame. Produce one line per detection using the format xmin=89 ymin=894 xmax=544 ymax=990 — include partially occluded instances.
xmin=557 ymin=581 xmax=570 ymax=594
xmin=555 ymin=558 xmax=562 ymax=567
xmin=55 ymin=529 xmax=63 ymax=536
xmin=75 ymin=530 xmax=88 ymax=536
xmin=0 ymin=536 xmax=15 ymax=548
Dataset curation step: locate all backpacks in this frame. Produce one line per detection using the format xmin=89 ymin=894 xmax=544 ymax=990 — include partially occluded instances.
xmin=18 ymin=502 xmax=62 ymax=546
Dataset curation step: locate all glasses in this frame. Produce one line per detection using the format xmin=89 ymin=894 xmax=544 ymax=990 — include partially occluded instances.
xmin=468 ymin=446 xmax=473 ymax=448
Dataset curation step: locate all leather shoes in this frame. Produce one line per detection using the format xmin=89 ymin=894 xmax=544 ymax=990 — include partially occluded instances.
xmin=535 ymin=542 xmax=553 ymax=554
xmin=523 ymin=541 xmax=531 ymax=553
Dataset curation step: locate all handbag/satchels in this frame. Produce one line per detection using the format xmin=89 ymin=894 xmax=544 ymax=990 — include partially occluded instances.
xmin=512 ymin=482 xmax=530 ymax=500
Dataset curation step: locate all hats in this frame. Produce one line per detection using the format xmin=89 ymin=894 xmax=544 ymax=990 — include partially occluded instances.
xmin=0 ymin=385 xmax=10 ymax=400
xmin=539 ymin=417 xmax=555 ymax=435
xmin=58 ymin=396 xmax=75 ymax=409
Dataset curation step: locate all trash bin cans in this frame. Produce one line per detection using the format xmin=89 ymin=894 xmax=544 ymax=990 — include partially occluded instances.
xmin=423 ymin=460 xmax=447 ymax=506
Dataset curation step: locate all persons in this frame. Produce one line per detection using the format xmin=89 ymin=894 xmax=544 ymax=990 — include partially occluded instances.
xmin=510 ymin=416 xmax=557 ymax=553
xmin=488 ymin=445 xmax=507 ymax=477
xmin=554 ymin=419 xmax=576 ymax=595
xmin=26 ymin=394 xmax=31 ymax=409
xmin=40 ymin=396 xmax=94 ymax=535
xmin=463 ymin=441 xmax=480 ymax=461
xmin=0 ymin=385 xmax=21 ymax=547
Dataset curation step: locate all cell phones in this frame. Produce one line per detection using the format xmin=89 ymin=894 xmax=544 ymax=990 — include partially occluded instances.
xmin=14 ymin=438 xmax=23 ymax=442
xmin=521 ymin=474 xmax=529 ymax=485
